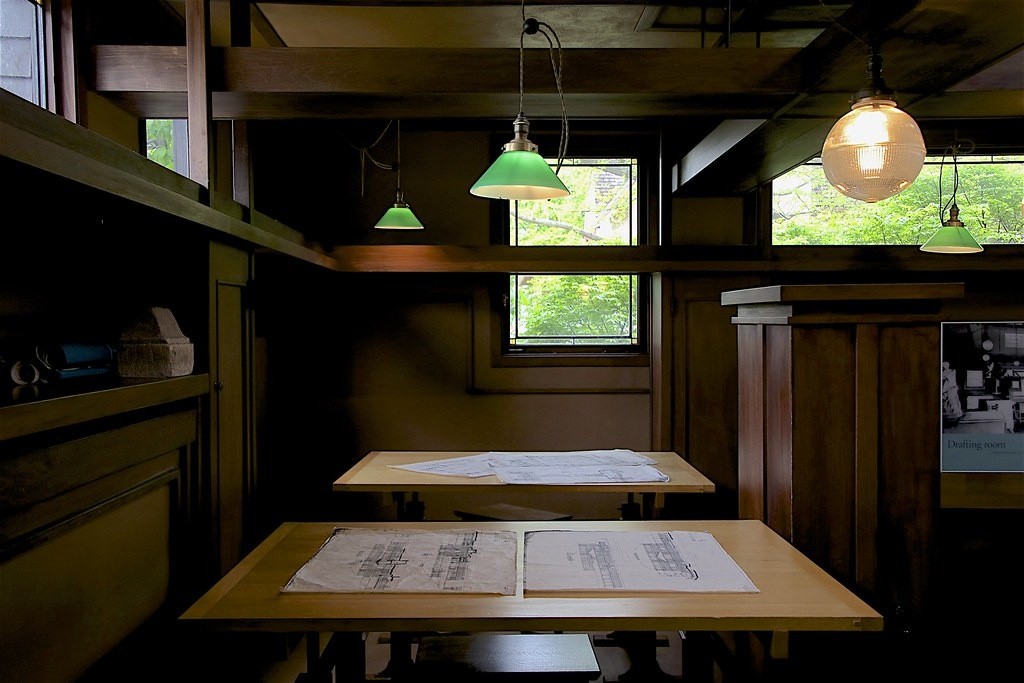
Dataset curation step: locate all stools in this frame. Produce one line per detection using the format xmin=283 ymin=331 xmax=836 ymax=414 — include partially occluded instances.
xmin=453 ymin=503 xmax=573 ymax=521
xmin=414 ymin=633 xmax=602 ymax=683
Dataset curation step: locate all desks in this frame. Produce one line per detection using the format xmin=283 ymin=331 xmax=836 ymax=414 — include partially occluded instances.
xmin=176 ymin=522 xmax=885 ymax=683
xmin=333 ymin=452 xmax=716 ymax=646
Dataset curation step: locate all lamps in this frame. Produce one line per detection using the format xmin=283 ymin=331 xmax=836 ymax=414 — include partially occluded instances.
xmin=374 ymin=118 xmax=424 ymax=229
xmin=920 ymin=139 xmax=985 ymax=253
xmin=821 ymin=27 xmax=927 ymax=203
xmin=469 ymin=18 xmax=570 ymax=200
